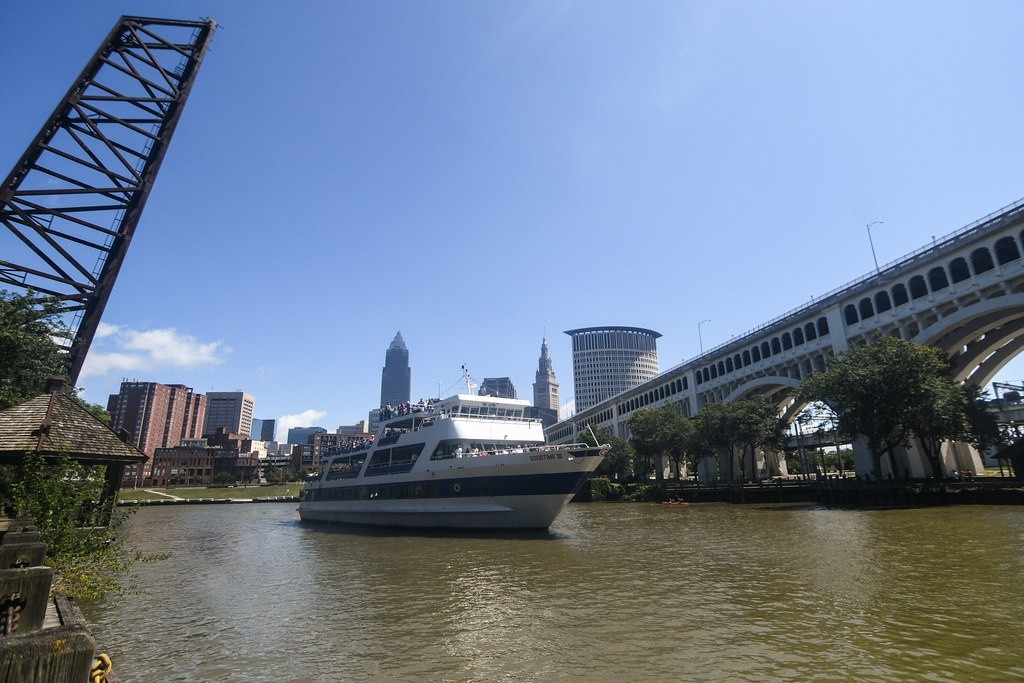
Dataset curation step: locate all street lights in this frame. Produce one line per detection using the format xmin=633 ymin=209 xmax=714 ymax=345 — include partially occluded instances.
xmin=864 ymin=218 xmax=885 ymax=275
xmin=698 ymin=317 xmax=711 ymax=356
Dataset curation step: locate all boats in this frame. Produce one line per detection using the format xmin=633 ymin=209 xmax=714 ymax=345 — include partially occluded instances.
xmin=297 ymin=363 xmax=614 ymax=533
xmin=662 ymin=502 xmax=689 ymax=505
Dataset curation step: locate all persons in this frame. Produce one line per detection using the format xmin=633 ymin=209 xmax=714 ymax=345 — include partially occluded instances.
xmin=324 ymin=398 xmax=581 ymax=459
